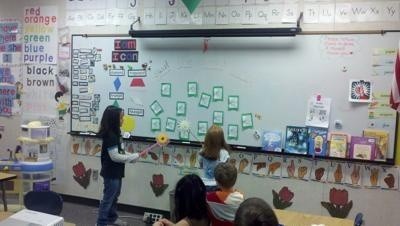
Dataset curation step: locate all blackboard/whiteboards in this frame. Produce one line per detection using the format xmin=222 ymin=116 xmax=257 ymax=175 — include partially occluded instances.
xmin=71 ymin=30 xmax=400 ymax=166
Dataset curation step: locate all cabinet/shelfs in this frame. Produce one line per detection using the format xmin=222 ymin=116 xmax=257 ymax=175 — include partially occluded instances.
xmin=16 ymin=123 xmax=55 ymax=193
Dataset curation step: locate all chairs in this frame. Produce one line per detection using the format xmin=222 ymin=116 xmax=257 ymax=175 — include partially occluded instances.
xmin=353 ymin=211 xmax=365 ymax=226
xmin=24 ymin=188 xmax=62 ymax=215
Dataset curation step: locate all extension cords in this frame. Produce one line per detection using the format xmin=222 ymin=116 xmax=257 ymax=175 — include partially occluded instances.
xmin=143 ymin=212 xmax=162 ymax=223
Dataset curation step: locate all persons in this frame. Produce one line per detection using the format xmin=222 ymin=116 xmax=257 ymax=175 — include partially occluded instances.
xmin=205 ymin=162 xmax=245 ymax=225
xmin=95 ymin=106 xmax=148 ymax=226
xmin=235 ymin=197 xmax=284 ymax=225
xmin=151 ymin=174 xmax=209 ymax=226
xmin=196 ymin=126 xmax=231 ymax=191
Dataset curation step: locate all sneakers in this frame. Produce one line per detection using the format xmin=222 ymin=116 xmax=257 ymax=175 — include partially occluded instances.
xmin=111 ymin=218 xmax=128 ymax=226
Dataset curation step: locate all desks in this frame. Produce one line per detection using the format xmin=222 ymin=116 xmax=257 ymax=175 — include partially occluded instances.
xmin=247 ymin=205 xmax=354 ymax=226
xmin=0 ymin=171 xmax=16 ymax=211
xmin=1 ymin=207 xmax=76 ymax=226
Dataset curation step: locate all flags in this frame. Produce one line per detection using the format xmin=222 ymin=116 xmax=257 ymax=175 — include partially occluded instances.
xmin=387 ymin=51 xmax=400 ymax=113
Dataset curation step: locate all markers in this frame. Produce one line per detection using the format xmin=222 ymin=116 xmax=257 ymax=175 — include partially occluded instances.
xmin=77 ymin=131 xmax=91 ymax=134
xmin=180 ymin=141 xmax=192 ymax=145
xmin=233 ymin=146 xmax=247 ymax=148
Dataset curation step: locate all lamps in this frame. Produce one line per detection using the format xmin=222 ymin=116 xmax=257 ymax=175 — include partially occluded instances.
xmin=128 ymin=10 xmax=304 ymax=38
